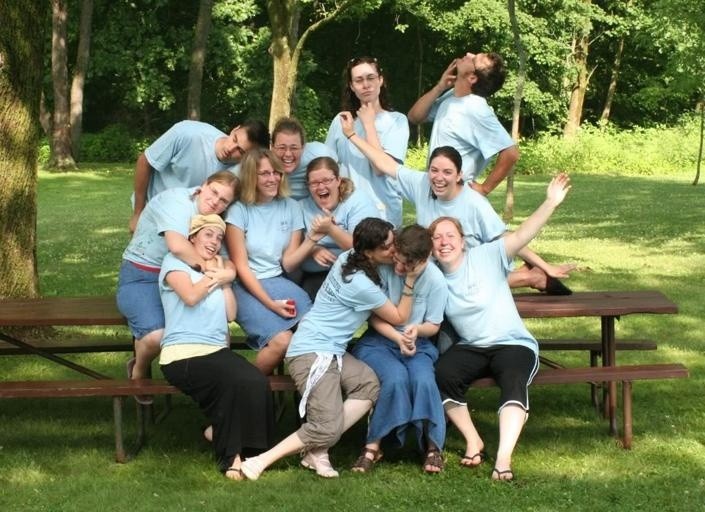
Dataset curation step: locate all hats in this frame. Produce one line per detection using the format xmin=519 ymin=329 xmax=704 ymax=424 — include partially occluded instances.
xmin=188 ymin=214 xmax=226 ymax=238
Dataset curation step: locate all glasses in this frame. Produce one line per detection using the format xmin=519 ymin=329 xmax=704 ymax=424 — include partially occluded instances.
xmin=272 ymin=144 xmax=304 ymax=152
xmin=350 ymin=73 xmax=381 ymax=83
xmin=207 ymin=184 xmax=231 ymax=208
xmin=307 ymin=176 xmax=338 ymax=186
xmin=472 ymin=53 xmax=477 ymax=72
xmin=256 ymin=170 xmax=281 ymax=177
xmin=431 ymin=232 xmax=462 ymax=241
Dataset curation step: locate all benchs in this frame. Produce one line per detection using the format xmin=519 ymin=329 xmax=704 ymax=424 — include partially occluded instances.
xmin=0 ymin=336 xmax=291 ymax=402
xmin=0 ymin=379 xmax=309 ymax=466
xmin=431 ymin=362 xmax=691 ymax=450
xmin=528 ymin=337 xmax=658 ymax=414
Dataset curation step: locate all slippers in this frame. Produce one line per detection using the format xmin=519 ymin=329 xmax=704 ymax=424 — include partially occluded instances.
xmin=543 ymin=270 xmax=573 ymax=297
xmin=491 ymin=468 xmax=514 ymax=481
xmin=460 ymin=450 xmax=487 ymax=468
xmin=126 ymin=357 xmax=155 ymax=405
xmin=225 ymin=464 xmax=244 ymax=481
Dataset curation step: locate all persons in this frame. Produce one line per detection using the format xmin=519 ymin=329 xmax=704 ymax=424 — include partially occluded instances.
xmin=323 ymin=55 xmax=410 ymax=234
xmin=338 ymin=110 xmax=578 ymax=279
xmin=116 ymin=170 xmax=243 ymax=406
xmin=238 ymin=216 xmax=395 ymax=481
xmin=271 ymin=117 xmax=339 ymax=201
xmin=297 ymin=156 xmax=378 ymax=305
xmin=204 ymin=147 xmax=337 ymax=442
xmin=427 ymin=170 xmax=572 ymax=482
xmin=351 ymin=224 xmax=447 ymax=474
xmin=406 ymin=51 xmax=573 ymax=295
xmin=128 ymin=119 xmax=270 ymax=236
xmin=157 ymin=213 xmax=268 ymax=482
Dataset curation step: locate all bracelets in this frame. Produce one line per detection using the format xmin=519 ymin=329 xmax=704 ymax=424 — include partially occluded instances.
xmin=402 ymin=292 xmax=414 ymax=297
xmin=403 ymin=280 xmax=414 ymax=290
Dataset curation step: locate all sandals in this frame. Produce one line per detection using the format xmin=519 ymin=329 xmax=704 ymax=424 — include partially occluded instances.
xmin=351 ymin=446 xmax=384 ymax=474
xmin=424 ymin=448 xmax=444 ymax=474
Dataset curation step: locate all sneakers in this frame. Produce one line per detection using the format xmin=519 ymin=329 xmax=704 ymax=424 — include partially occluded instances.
xmin=300 ymin=451 xmax=339 ymax=478
xmin=240 ymin=457 xmax=262 ymax=482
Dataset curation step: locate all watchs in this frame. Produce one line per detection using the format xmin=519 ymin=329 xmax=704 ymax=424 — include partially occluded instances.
xmin=346 ymin=132 xmax=356 ymax=139
